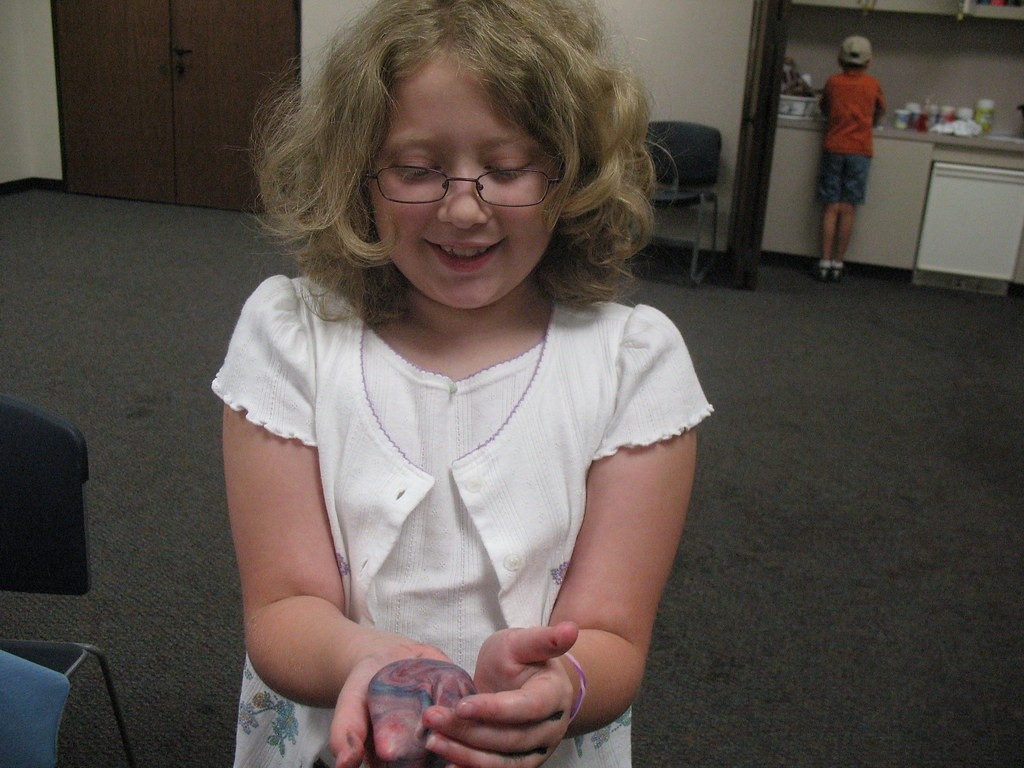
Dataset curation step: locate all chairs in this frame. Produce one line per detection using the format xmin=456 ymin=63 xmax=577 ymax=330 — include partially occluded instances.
xmin=644 ymin=121 xmax=722 ymax=285
xmin=0 ymin=397 xmax=135 ymax=768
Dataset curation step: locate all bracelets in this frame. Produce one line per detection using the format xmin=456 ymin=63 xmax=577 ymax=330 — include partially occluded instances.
xmin=554 ymin=652 xmax=588 ymax=731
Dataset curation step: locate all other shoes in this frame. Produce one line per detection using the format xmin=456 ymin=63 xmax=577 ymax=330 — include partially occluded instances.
xmin=819 ymin=259 xmax=843 ymax=280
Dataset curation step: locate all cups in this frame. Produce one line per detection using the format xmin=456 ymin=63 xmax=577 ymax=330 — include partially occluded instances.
xmin=893 ymin=99 xmax=994 ymax=137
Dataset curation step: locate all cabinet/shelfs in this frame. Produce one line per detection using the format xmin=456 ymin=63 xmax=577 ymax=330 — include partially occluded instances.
xmin=762 ymin=128 xmax=1024 ymax=296
xmin=792 ymin=0 xmax=1024 ymax=20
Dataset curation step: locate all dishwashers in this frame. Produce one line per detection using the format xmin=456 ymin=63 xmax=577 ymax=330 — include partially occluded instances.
xmin=912 ymin=162 xmax=1024 ymax=297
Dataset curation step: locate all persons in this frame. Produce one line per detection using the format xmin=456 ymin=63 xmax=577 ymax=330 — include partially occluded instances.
xmin=212 ymin=1 xmax=715 ymax=768
xmin=818 ymin=34 xmax=888 ymax=284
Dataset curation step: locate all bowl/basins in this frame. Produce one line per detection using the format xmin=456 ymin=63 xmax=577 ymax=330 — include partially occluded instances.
xmin=778 ymin=94 xmax=821 ymax=116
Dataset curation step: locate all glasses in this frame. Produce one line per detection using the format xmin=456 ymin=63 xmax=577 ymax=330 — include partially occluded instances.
xmin=368 ymin=165 xmax=562 ymax=206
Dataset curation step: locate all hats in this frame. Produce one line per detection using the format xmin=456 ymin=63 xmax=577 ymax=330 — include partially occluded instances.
xmin=840 ymin=36 xmax=871 ymax=66
